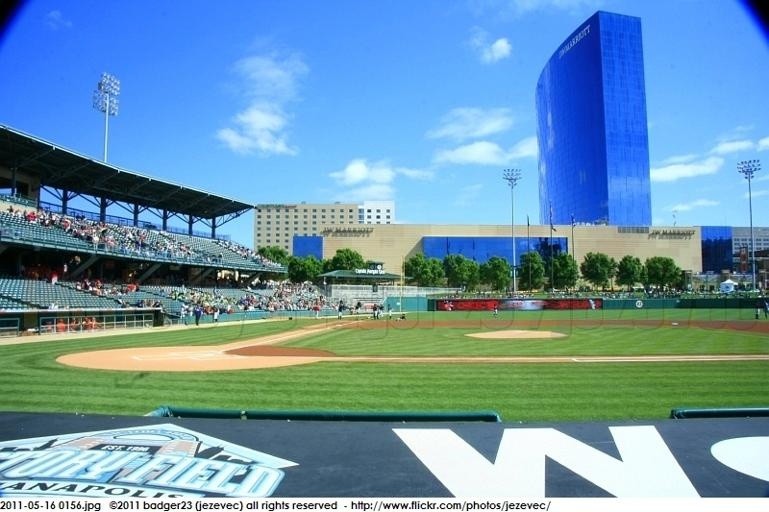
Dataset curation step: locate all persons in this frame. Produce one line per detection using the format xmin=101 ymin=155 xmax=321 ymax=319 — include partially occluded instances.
xmin=493 ymin=303 xmax=498 ymax=315
xmin=443 ymin=299 xmax=449 ymax=310
xmin=438 ymin=288 xmax=769 ymax=300
xmin=754 ymin=299 xmax=760 ymax=319
xmin=387 ymin=303 xmax=393 ymax=320
xmin=0 ymin=193 xmax=386 ymax=335
xmin=395 ymin=312 xmax=408 ymax=322
xmin=762 ymin=299 xmax=769 ymax=320
xmin=447 ymin=300 xmax=454 ymax=311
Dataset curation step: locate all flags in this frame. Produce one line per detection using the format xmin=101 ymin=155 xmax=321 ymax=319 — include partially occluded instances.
xmin=550 ymin=204 xmax=558 ymax=232
